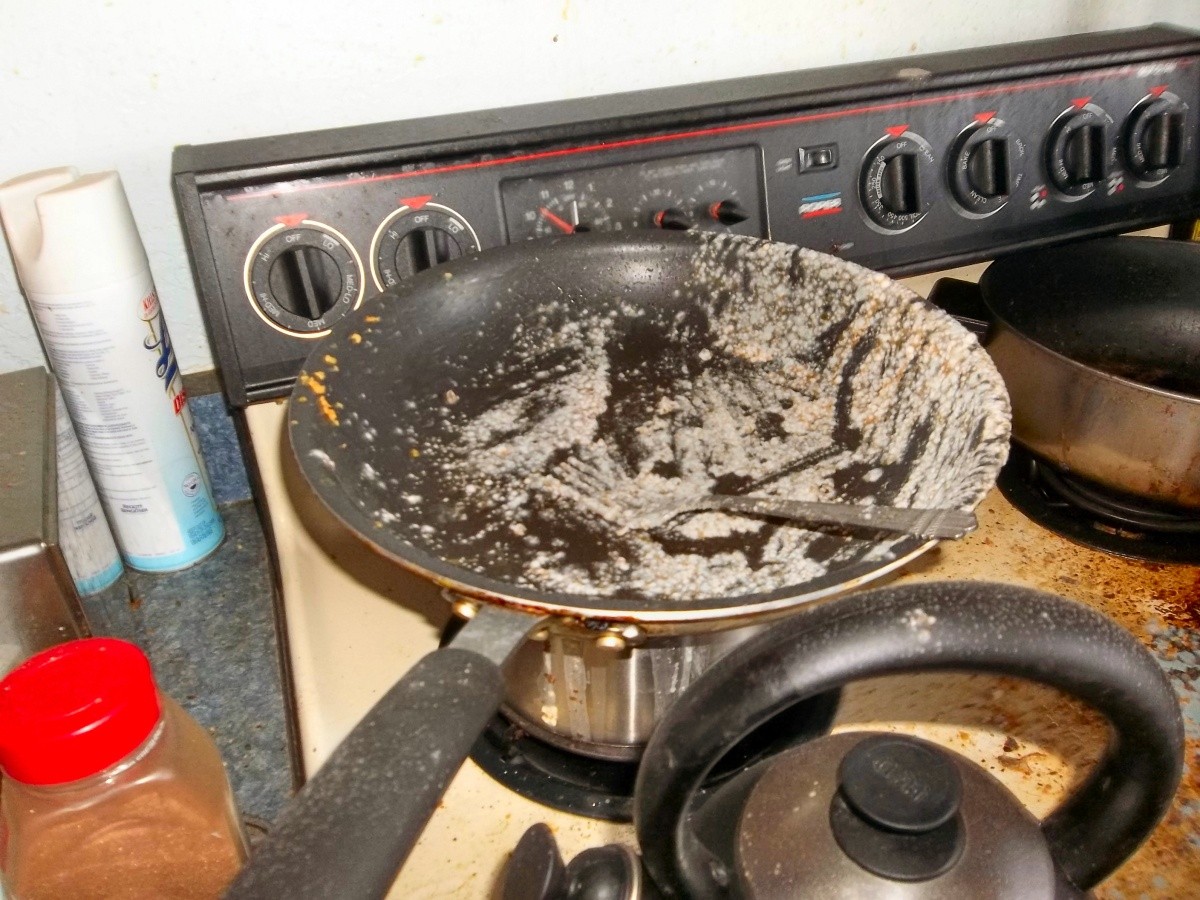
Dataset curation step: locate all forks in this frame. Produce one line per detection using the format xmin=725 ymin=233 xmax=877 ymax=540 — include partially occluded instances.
xmin=538 ymin=454 xmax=980 ymax=541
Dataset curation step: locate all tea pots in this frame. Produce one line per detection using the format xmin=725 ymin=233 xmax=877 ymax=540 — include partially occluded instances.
xmin=497 ymin=583 xmax=1186 ymax=900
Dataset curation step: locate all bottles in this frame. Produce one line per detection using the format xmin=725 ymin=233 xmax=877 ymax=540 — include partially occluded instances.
xmin=0 ymin=164 xmax=228 ymax=577
xmin=1 ymin=636 xmax=253 ymax=900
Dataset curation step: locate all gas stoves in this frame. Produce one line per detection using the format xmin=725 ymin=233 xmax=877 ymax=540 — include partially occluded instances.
xmin=169 ymin=21 xmax=1200 ymax=900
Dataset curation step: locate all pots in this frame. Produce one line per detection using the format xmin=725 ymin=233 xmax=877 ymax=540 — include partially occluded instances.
xmin=215 ymin=226 xmax=1015 ymax=900
xmin=925 ymin=235 xmax=1200 ymax=509
